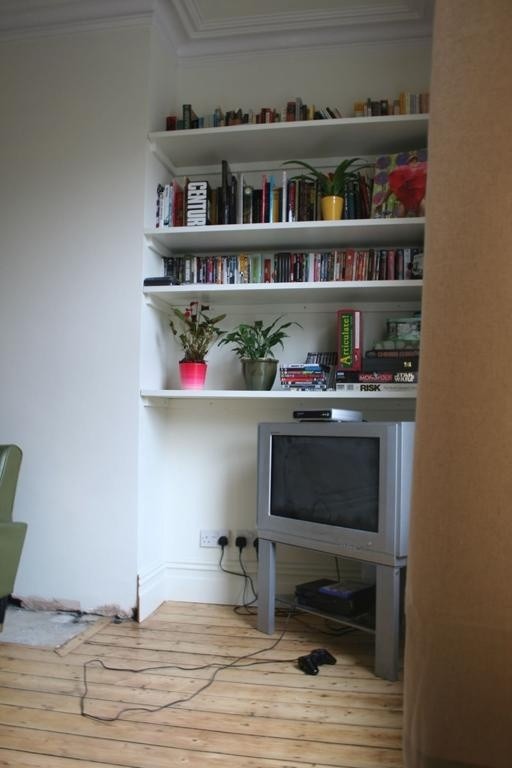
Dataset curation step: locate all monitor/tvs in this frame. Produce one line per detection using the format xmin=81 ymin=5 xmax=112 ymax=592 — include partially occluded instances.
xmin=156 ymin=146 xmax=427 ymax=224
xmin=279 ymin=338 xmax=420 ymax=391
xmin=164 ymin=92 xmax=428 ymax=132
xmin=161 ymin=248 xmax=424 ymax=285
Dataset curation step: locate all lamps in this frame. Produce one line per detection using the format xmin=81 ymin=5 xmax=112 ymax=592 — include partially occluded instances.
xmin=0 ymin=444 xmax=28 ymax=630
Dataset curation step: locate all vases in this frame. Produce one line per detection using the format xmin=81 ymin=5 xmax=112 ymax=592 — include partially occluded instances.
xmin=169 ymin=298 xmax=226 ymax=362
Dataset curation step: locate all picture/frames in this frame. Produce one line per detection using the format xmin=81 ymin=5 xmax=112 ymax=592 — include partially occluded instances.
xmin=254 ymin=535 xmax=406 ymax=683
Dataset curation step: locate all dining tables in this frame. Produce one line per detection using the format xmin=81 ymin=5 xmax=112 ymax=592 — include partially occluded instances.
xmin=216 ymin=314 xmax=304 ymax=390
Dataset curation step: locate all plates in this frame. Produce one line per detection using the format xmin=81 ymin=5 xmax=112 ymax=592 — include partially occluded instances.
xmin=140 ymin=114 xmax=432 ymax=401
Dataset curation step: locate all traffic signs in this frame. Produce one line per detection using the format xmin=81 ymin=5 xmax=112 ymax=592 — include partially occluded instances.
xmin=199 ymin=530 xmax=230 ymax=546
xmin=236 ymin=530 xmax=259 ymax=546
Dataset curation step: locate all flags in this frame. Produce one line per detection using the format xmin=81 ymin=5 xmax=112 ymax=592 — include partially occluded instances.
xmin=178 ymin=361 xmax=208 ymax=391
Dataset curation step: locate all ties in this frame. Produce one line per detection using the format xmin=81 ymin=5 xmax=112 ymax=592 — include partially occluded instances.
xmin=256 ymin=421 xmax=416 ymax=559
